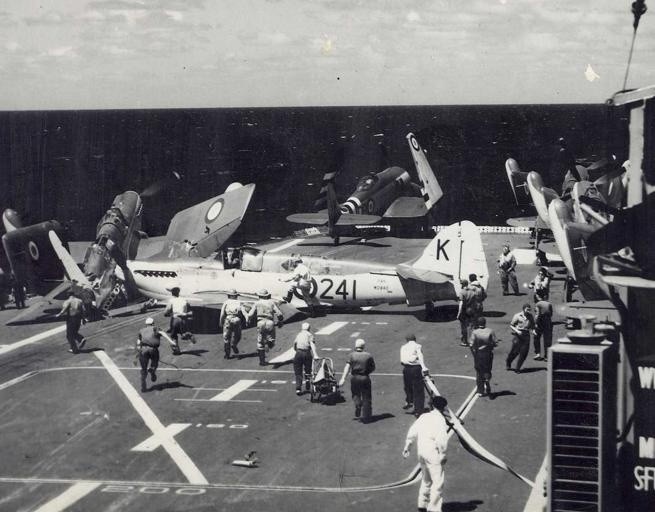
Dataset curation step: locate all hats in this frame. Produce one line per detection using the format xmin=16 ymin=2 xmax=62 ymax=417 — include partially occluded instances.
xmin=255 ymin=289 xmax=270 ymax=296
xmin=227 ymin=289 xmax=238 ymax=295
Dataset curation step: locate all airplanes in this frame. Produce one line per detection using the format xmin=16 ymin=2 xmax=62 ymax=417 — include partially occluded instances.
xmin=285 ymin=131 xmax=444 ymax=246
xmin=108 ymin=218 xmax=489 ymax=326
xmin=502 ymin=148 xmax=654 ymax=298
xmin=2 ymin=182 xmax=257 ymax=324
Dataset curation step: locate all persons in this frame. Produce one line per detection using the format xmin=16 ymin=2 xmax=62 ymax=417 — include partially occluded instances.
xmin=467 ymin=273 xmax=487 ymax=329
xmin=218 ymin=288 xmax=251 ymax=359
xmin=505 ymin=303 xmax=538 ymax=373
xmin=0 ymin=268 xmax=9 ymax=310
xmin=469 ymin=316 xmax=498 ymax=397
xmin=136 ymin=317 xmax=177 ymax=392
xmin=532 ymin=288 xmax=553 ymax=362
xmin=292 ymin=322 xmax=321 ymax=395
xmin=496 ymin=246 xmax=521 ymax=296
xmin=527 ymin=267 xmax=552 ymax=314
xmin=337 ymin=337 xmax=376 ymax=424
xmin=246 ymin=288 xmax=284 ymax=366
xmin=457 ymin=279 xmax=478 ymax=346
xmin=163 ymin=286 xmax=197 ymax=356
xmin=399 ymin=333 xmax=430 ymax=418
xmin=9 ymin=268 xmax=29 ymax=310
xmin=55 ymin=290 xmax=89 ymax=353
xmin=401 ymin=396 xmax=450 ymax=512
xmin=279 ymin=256 xmax=317 ymax=318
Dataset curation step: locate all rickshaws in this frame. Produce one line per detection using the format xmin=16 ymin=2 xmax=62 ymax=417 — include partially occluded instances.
xmin=308 ymin=357 xmax=340 ymax=402
